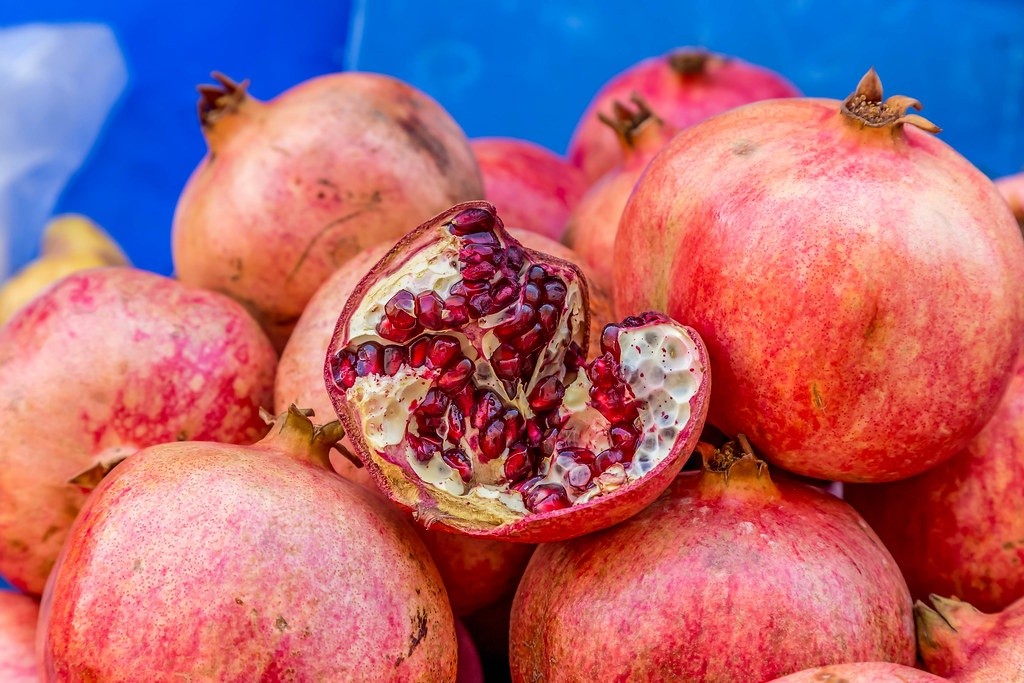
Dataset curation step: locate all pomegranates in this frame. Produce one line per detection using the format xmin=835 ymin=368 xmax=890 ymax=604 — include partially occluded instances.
xmin=0 ymin=47 xmax=1024 ymax=683
